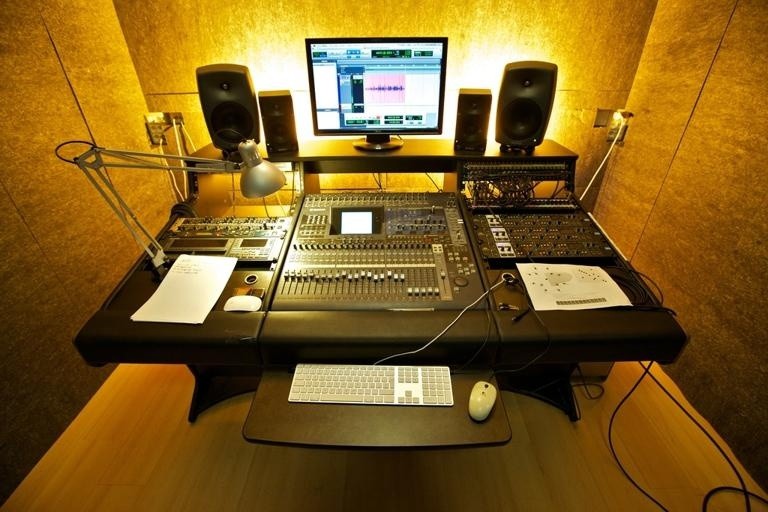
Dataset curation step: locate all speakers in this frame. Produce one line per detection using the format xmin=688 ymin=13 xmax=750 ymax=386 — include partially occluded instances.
xmin=494 ymin=60 xmax=558 ymax=154
xmin=195 ymin=64 xmax=260 ymax=151
xmin=258 ymin=89 xmax=299 ymax=154
xmin=454 ymin=88 xmax=492 ymax=154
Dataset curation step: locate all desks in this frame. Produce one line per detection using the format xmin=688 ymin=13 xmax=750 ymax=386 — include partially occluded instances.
xmin=72 ymin=139 xmax=688 ymax=450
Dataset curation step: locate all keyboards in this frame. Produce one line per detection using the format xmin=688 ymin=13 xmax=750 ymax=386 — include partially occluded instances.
xmin=288 ymin=363 xmax=454 ymax=406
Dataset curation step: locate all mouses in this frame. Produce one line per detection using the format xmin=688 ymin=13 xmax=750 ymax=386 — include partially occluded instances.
xmin=468 ymin=380 xmax=497 ymax=421
xmin=223 ymin=295 xmax=262 ymax=311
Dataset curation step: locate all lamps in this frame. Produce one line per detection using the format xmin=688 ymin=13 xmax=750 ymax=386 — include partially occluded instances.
xmin=55 ymin=139 xmax=286 ymax=268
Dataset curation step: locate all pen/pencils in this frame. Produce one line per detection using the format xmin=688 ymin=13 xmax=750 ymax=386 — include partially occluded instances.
xmin=511 ymin=307 xmax=529 ymax=322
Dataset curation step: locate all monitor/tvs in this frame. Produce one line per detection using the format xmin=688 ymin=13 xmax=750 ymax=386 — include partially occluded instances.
xmin=305 ymin=37 xmax=448 ymax=152
